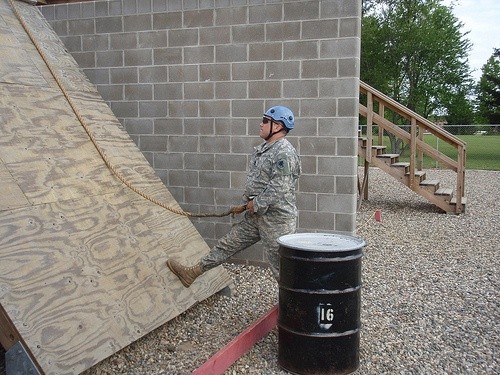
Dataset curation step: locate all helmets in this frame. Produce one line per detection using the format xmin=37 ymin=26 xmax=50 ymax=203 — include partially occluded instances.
xmin=264 ymin=106 xmax=295 ymax=129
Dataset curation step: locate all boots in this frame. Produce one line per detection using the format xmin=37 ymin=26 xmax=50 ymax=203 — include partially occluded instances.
xmin=168 ymin=257 xmax=206 ymax=288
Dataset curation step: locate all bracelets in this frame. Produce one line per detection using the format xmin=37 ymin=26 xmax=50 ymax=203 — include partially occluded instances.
xmin=243 ymin=205 xmax=247 ymax=211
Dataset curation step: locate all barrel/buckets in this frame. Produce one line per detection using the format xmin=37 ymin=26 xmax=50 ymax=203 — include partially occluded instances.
xmin=275 ymin=233 xmax=366 ymax=375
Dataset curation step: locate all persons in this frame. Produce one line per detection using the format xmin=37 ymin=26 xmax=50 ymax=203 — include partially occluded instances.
xmin=166 ymin=105 xmax=302 ymax=288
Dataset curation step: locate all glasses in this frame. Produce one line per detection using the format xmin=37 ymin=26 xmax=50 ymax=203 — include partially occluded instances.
xmin=262 ymin=119 xmax=273 ymax=124
